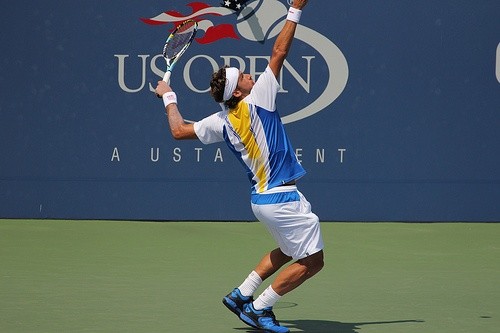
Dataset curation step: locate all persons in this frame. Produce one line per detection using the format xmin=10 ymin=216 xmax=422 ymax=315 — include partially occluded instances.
xmin=154 ymin=0 xmax=326 ymax=333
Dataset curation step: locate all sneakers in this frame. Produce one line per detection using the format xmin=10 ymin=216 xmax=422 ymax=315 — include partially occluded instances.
xmin=239 ymin=301 xmax=290 ymax=333
xmin=222 ymin=287 xmax=255 ymax=317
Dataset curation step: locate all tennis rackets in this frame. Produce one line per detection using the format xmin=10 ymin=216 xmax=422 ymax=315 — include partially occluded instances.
xmin=157 ymin=20 xmax=198 ymax=98
